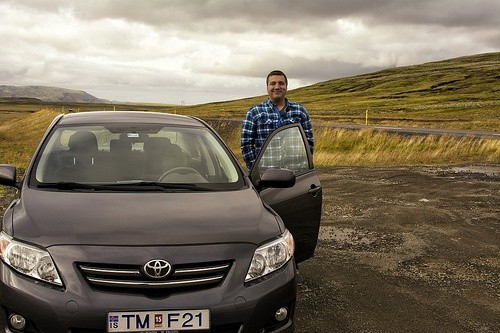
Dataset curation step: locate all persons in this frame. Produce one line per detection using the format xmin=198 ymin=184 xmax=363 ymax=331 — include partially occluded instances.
xmin=241 ymin=71 xmax=314 ymax=275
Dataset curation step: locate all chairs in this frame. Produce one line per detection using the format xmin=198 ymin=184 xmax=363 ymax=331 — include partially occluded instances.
xmin=131 ymin=137 xmax=190 ymax=183
xmin=57 ymin=130 xmax=114 ymax=182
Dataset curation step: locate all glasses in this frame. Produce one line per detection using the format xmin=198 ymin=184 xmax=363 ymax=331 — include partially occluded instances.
xmin=281 ymin=106 xmax=292 ymax=117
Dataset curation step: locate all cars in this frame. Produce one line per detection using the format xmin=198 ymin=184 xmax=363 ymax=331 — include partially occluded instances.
xmin=0 ymin=111 xmax=323 ymax=333
